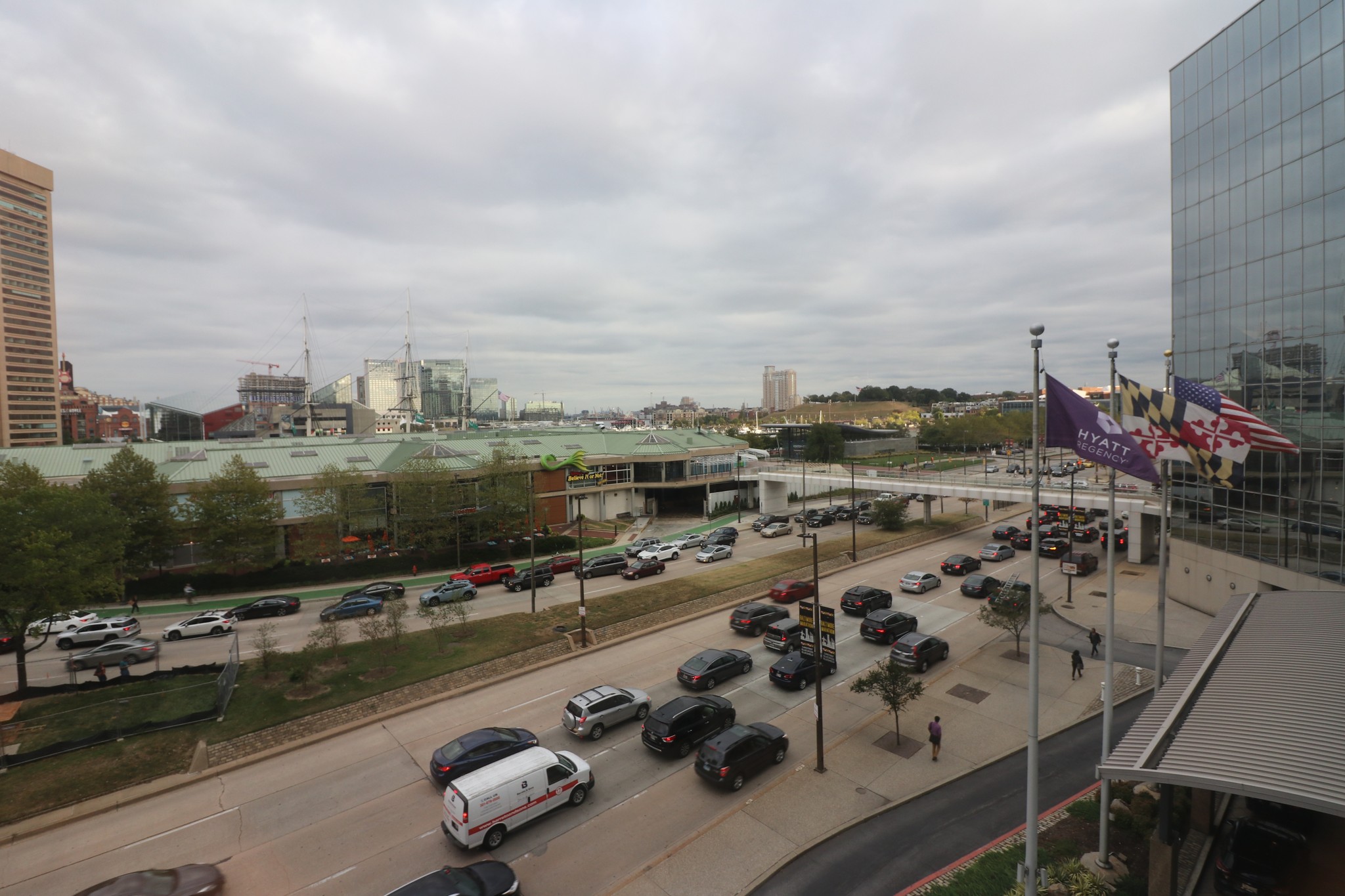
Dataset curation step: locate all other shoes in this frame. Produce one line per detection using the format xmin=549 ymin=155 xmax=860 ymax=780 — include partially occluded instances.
xmin=932 ymin=757 xmax=937 ymax=760
xmin=1079 ymin=674 xmax=1082 ymax=677
xmin=1072 ymin=678 xmax=1075 ymax=680
xmin=1090 ymin=655 xmax=1093 ymax=657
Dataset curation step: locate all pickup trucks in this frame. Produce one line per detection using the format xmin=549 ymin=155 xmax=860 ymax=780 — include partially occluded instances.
xmin=449 ymin=563 xmax=515 ymax=586
xmin=988 ymin=581 xmax=1032 ymax=616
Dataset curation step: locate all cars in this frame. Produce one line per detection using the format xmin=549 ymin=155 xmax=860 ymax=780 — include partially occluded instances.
xmin=898 ymin=571 xmax=941 ymax=594
xmin=941 ymin=554 xmax=982 ymax=576
xmin=340 ymin=581 xmax=405 ymax=602
xmin=229 ymin=595 xmax=301 ymax=621
xmin=978 ymin=504 xmax=1138 ymax=579
xmin=768 ymin=579 xmax=815 ymax=605
xmin=0 ymin=591 xmax=238 ymax=670
xmin=909 ymin=449 xmax=1173 ymax=504
xmin=960 ymin=573 xmax=1000 ymax=599
xmin=67 ymin=863 xmax=227 ymax=896
xmin=622 ymin=487 xmax=917 ymax=587
xmin=677 ymin=648 xmax=753 ymax=691
xmin=319 ymin=593 xmax=383 ymax=622
xmin=769 ymin=650 xmax=838 ymax=690
xmin=536 ymin=555 xmax=580 ymax=575
xmin=381 ymin=859 xmax=518 ymax=896
xmin=430 ymin=727 xmax=539 ymax=785
xmin=419 ymin=579 xmax=477 ymax=607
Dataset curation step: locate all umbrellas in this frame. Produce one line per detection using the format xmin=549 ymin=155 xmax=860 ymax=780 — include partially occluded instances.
xmin=317 ymin=531 xmax=416 ymax=557
xmin=489 ymin=513 xmax=542 ymax=540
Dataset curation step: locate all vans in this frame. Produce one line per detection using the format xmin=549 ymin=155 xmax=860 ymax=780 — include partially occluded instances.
xmin=574 ymin=553 xmax=629 ymax=580
xmin=441 ymin=746 xmax=595 ymax=854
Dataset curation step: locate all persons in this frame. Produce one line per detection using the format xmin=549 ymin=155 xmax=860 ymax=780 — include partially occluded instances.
xmin=119 ymin=658 xmax=130 ymax=678
xmin=899 ymin=463 xmax=905 ymax=470
xmin=1088 ymin=628 xmax=1101 ymax=658
xmin=928 ymin=716 xmax=943 ymax=762
xmin=131 ymin=596 xmax=140 ymax=614
xmin=183 ymin=583 xmax=195 ymax=606
xmin=96 ymin=660 xmax=108 ymax=684
xmin=1013 ymin=468 xmax=1031 ymax=478
xmin=1070 ymin=650 xmax=1083 ymax=681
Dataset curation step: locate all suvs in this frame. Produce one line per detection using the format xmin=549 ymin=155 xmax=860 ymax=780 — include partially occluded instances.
xmin=729 ymin=602 xmax=789 ymax=637
xmin=503 ymin=565 xmax=555 ymax=592
xmin=841 ymin=585 xmax=892 ymax=618
xmin=763 ymin=618 xmax=805 ymax=655
xmin=562 ymin=684 xmax=652 ymax=741
xmin=641 ymin=694 xmax=736 ymax=760
xmin=859 ymin=608 xmax=918 ymax=645
xmin=694 ymin=721 xmax=790 ymax=793
xmin=889 ymin=631 xmax=949 ymax=674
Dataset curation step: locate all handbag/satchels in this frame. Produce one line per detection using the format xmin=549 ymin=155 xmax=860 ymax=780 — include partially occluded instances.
xmin=1077 ymin=663 xmax=1084 ymax=669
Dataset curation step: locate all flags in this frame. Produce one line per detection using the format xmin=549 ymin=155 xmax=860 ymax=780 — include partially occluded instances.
xmin=498 ymin=391 xmax=510 ymax=403
xmin=1171 ymin=374 xmax=1302 ymax=459
xmin=1044 ymin=373 xmax=1164 ymax=489
xmin=1117 ymin=372 xmax=1254 ymax=493
xmin=414 ymin=412 xmax=426 ymax=424
xmin=468 ymin=420 xmax=479 ymax=431
